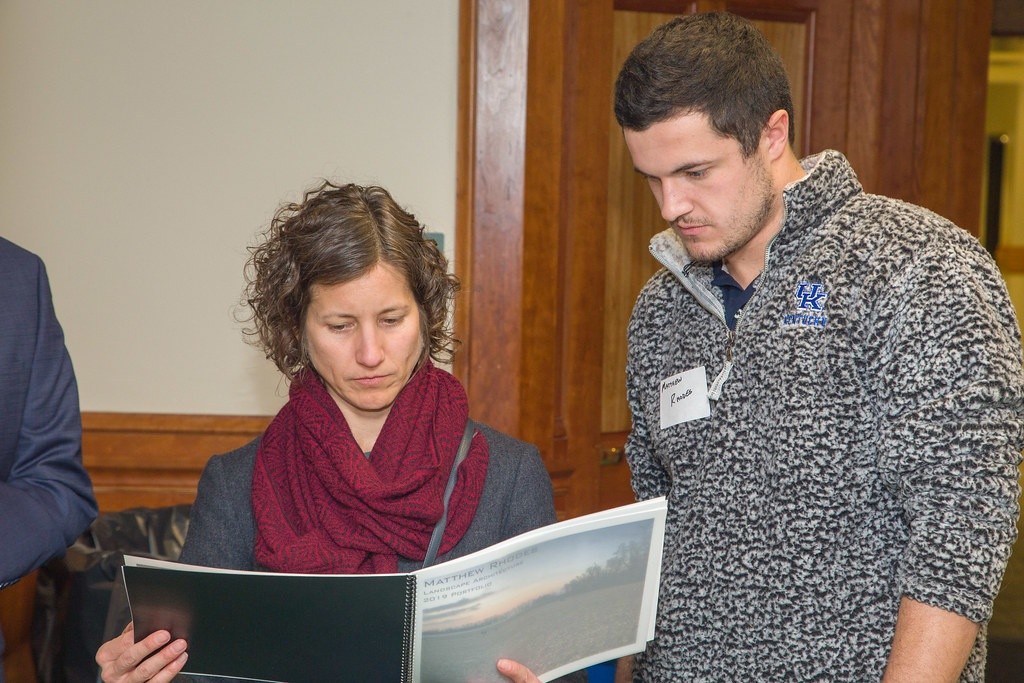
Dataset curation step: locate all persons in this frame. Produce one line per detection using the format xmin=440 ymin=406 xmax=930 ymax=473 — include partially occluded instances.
xmin=612 ymin=10 xmax=1024 ymax=683
xmin=0 ymin=234 xmax=100 ymax=590
xmin=94 ymin=182 xmax=588 ymax=683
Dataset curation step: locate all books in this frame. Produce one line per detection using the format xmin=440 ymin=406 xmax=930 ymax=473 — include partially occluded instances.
xmin=120 ymin=493 xmax=667 ymax=683
